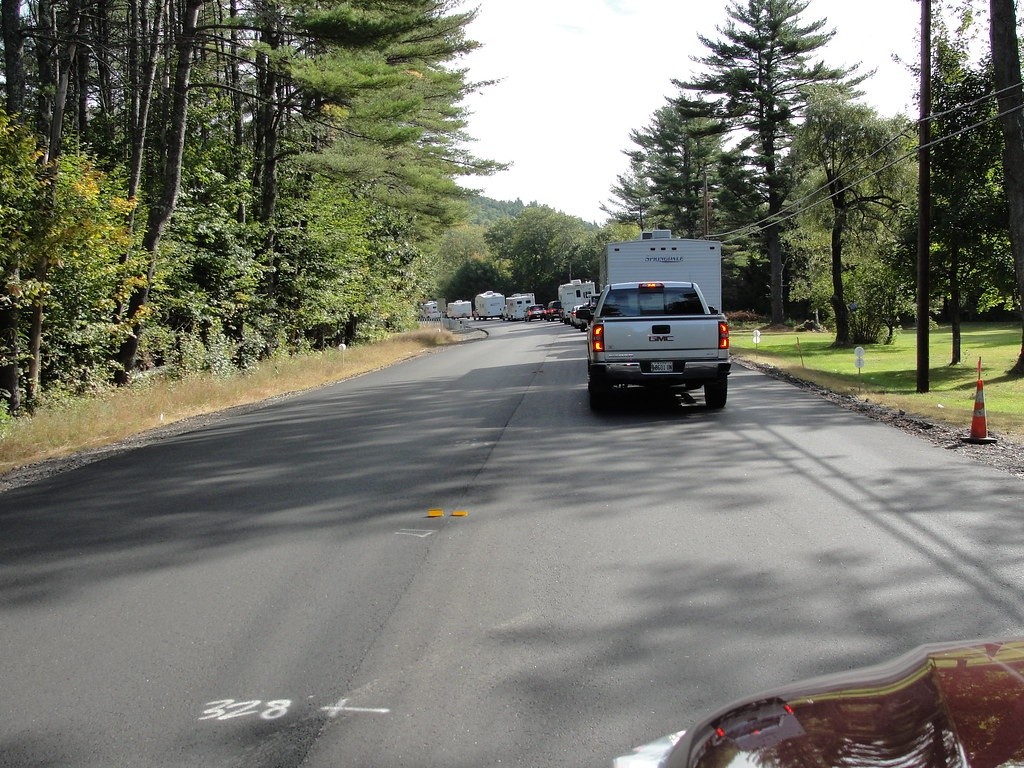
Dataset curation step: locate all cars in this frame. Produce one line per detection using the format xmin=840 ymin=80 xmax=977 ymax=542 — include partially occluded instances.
xmin=563 ymin=293 xmax=601 ymax=332
xmin=656 ymin=631 xmax=1024 ymax=768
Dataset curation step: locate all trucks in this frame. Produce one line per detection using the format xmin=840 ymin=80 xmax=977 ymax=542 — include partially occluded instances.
xmin=598 ymin=229 xmax=723 ymax=313
xmin=472 ymin=290 xmax=505 ymax=320
xmin=423 ymin=300 xmax=438 ymax=313
xmin=446 ymin=299 xmax=472 ymax=320
xmin=557 ymin=279 xmax=595 ymax=314
xmin=504 ymin=292 xmax=536 ymax=322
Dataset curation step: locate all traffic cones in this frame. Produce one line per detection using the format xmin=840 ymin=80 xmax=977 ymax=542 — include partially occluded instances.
xmin=960 ymin=379 xmax=998 ymax=444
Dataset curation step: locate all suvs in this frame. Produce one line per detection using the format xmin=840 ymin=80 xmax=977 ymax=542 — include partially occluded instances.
xmin=523 ymin=304 xmax=547 ymax=322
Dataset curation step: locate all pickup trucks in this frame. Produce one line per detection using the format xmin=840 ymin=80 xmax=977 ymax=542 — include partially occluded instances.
xmin=545 ymin=301 xmax=564 ymax=320
xmin=574 ymin=279 xmax=736 ymax=414
